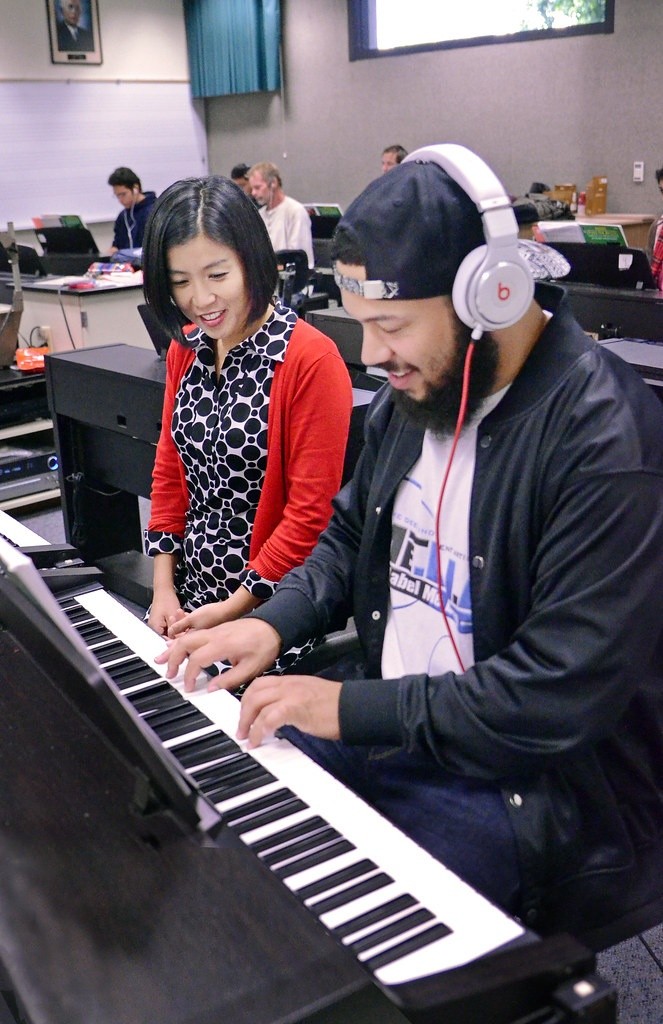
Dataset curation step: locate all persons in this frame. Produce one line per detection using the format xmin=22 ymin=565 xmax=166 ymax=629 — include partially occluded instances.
xmin=644 ymin=167 xmax=663 ymax=292
xmin=144 ymin=175 xmax=353 ymax=700
xmin=108 ymin=167 xmax=158 ymax=253
xmin=381 ymin=145 xmax=409 ymax=173
xmin=231 ymin=163 xmax=252 ymax=194
xmin=56 ymin=0 xmax=93 ymax=51
xmin=248 ymin=161 xmax=315 ymax=311
xmin=154 ymin=143 xmax=663 ymax=918
xmin=510 ymin=198 xmax=540 ymax=241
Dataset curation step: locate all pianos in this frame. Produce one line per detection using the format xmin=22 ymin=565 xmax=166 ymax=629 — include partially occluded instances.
xmin=302 ymin=203 xmax=342 ymax=267
xmin=44 ymin=345 xmax=383 ymax=607
xmin=0 ymin=512 xmax=619 ymax=1024
xmin=306 ymin=307 xmax=387 ymax=392
xmin=596 ymin=338 xmax=663 ymax=406
xmin=535 ymin=242 xmax=663 ymax=343
xmin=33 ymin=214 xmax=109 ymax=275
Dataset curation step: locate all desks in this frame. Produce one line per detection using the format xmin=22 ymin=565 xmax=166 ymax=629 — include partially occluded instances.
xmin=6 ymin=278 xmax=156 ymax=352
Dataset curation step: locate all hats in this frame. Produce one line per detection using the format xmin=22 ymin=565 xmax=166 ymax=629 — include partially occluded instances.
xmin=330 ymin=161 xmax=568 ymax=301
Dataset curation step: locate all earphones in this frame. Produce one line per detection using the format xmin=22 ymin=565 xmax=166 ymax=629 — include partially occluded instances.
xmin=133 ymin=188 xmax=138 ymax=195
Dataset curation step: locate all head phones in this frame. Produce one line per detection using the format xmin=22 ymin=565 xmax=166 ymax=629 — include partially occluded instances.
xmin=402 ymin=144 xmax=534 ymax=339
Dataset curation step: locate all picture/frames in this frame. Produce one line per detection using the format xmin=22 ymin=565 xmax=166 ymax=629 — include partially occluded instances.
xmin=47 ymin=0 xmax=103 ymax=66
